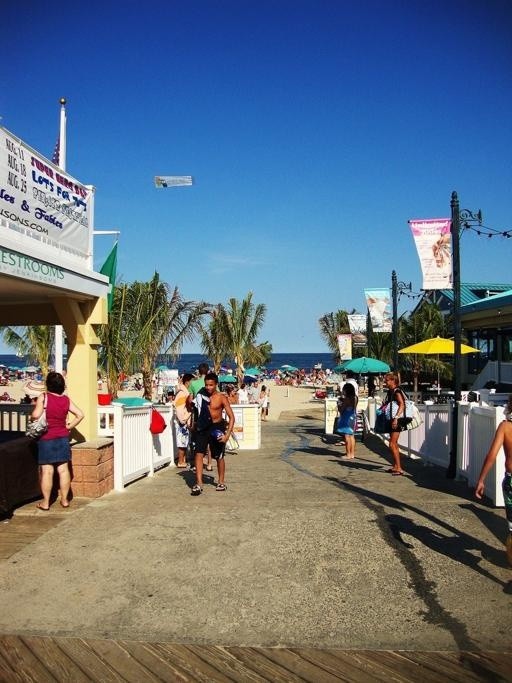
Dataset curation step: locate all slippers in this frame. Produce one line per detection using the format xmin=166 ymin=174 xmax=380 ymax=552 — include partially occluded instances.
xmin=385 ymin=469 xmax=403 ymax=475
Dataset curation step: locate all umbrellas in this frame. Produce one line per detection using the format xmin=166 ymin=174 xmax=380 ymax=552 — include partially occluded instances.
xmin=339 ymin=356 xmax=391 ymax=376
xmin=398 ymin=335 xmax=481 ymax=392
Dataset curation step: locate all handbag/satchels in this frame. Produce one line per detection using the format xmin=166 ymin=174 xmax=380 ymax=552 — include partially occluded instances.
xmin=24 ymin=392 xmax=49 ymax=440
xmin=374 ymin=387 xmax=424 ymax=434
xmin=150 ymin=404 xmax=167 ymax=434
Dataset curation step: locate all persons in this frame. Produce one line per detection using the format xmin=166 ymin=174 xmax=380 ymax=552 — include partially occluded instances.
xmin=169 ymin=363 xmax=270 ymax=423
xmin=474 ymin=394 xmax=511 ymax=568
xmin=173 ymin=373 xmax=196 ymax=468
xmin=364 ymin=373 xmax=378 ymax=397
xmin=186 ymin=363 xmax=220 ymax=471
xmin=263 ymin=367 xmax=326 ymax=383
xmin=192 ymin=372 xmax=235 ymax=495
xmin=382 ymin=369 xmax=407 ymax=476
xmin=31 ymin=371 xmax=85 ymax=511
xmin=335 ymin=382 xmax=359 ymax=459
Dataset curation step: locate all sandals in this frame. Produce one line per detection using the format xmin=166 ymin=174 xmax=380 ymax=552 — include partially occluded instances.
xmin=177 ymin=463 xmax=227 ymax=493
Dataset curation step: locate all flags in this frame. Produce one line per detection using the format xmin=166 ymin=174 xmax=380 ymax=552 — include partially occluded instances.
xmin=347 ymin=314 xmax=368 ymax=348
xmin=53 ymin=97 xmax=71 ymax=168
xmin=362 ymin=287 xmax=392 ymax=333
xmin=336 ymin=333 xmax=353 ymax=361
xmin=407 ymin=217 xmax=455 ymax=291
